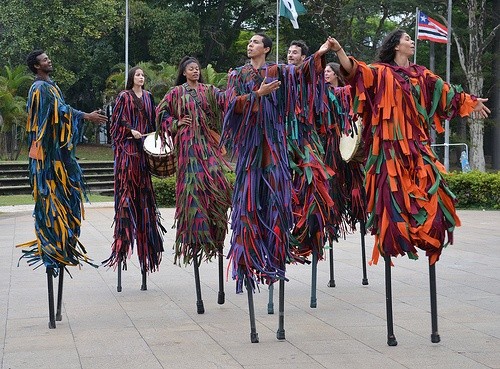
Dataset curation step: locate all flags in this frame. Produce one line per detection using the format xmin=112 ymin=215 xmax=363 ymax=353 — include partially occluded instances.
xmin=278 ymin=0 xmax=307 ymax=29
xmin=417 ymin=10 xmax=452 ymax=44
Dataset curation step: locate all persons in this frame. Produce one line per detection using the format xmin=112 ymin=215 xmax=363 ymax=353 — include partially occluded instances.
xmin=327 ymin=28 xmax=491 ymax=256
xmin=324 ymin=61 xmax=366 ymax=216
xmin=226 ymin=34 xmax=331 ymax=266
xmin=26 ymin=48 xmax=108 ymax=273
xmin=111 ymin=66 xmax=163 ymax=253
xmin=154 ymin=55 xmax=233 ymax=242
xmin=285 ymin=39 xmax=336 ymax=241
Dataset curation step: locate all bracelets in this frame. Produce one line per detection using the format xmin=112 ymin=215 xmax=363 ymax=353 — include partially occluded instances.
xmin=334 ymin=45 xmax=342 ymax=51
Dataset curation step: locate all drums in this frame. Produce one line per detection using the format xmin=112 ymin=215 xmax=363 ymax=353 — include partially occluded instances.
xmin=337 ymin=113 xmax=369 ymax=163
xmin=143 ymin=131 xmax=177 ymax=178
